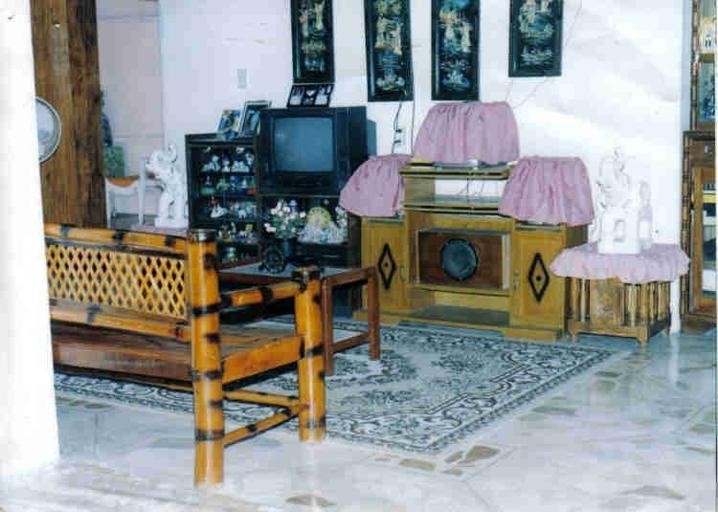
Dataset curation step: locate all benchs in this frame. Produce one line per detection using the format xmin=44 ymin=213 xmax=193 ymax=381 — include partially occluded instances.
xmin=42 ymin=218 xmax=329 ymax=486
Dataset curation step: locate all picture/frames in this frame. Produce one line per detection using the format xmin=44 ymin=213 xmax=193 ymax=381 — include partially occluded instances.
xmin=286 ymin=1 xmax=563 ymax=110
xmin=238 ymin=100 xmax=272 ymax=138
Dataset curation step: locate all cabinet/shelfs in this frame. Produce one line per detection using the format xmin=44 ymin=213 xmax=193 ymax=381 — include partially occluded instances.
xmin=400 ymin=162 xmax=513 ymax=296
xmin=359 ymin=219 xmax=407 ymax=313
xmin=517 ymin=232 xmax=568 ymax=326
xmin=255 ymin=193 xmax=357 ymax=315
xmin=186 ymin=136 xmax=255 ymax=304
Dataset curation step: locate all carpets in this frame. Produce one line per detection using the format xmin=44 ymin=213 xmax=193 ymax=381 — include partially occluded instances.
xmin=52 ymin=314 xmax=636 ymax=459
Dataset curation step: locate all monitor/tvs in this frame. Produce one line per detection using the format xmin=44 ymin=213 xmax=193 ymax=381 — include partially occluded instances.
xmin=257 ymin=105 xmax=368 ymax=196
